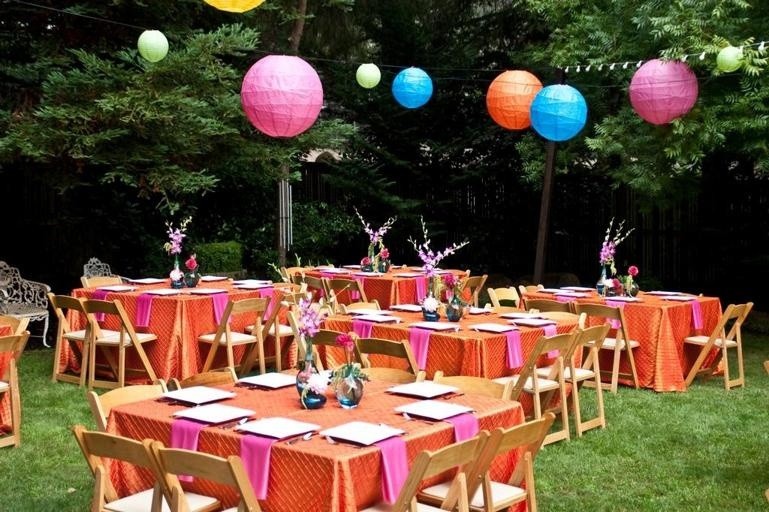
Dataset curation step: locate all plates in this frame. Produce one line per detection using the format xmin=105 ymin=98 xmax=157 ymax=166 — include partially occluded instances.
xmin=542 ymin=284 xmax=698 ymax=304
xmin=239 ymin=416 xmax=317 ymax=440
xmin=98 ymin=276 xmax=275 ymax=296
xmin=161 ymin=385 xmax=235 ymax=405
xmin=396 ymin=399 xmax=475 ymax=421
xmin=324 ymin=419 xmax=406 ymax=445
xmin=321 ymin=261 xmax=446 ymax=277
xmin=344 ymin=304 xmax=558 ymax=333
xmin=237 ymin=371 xmax=301 ymax=389
xmin=386 ymin=382 xmax=460 ymax=400
xmin=171 ymin=404 xmax=254 ymax=423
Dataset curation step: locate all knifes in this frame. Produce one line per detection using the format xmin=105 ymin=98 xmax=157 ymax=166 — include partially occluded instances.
xmin=284 ymin=432 xmax=319 ymax=446
xmin=218 ymin=417 xmax=256 ymax=429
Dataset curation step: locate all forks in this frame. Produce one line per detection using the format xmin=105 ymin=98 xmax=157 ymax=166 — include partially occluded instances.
xmin=402 ymin=412 xmax=434 ymax=426
xmin=326 ymin=436 xmax=360 ymax=449
xmin=440 ymin=393 xmax=465 ymax=400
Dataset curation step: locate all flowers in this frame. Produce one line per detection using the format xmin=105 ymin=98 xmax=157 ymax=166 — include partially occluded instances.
xmin=351 ymin=204 xmax=398 ymax=266
xmin=406 ymin=212 xmax=472 ymax=293
xmin=161 ymin=216 xmax=194 ymax=258
xmin=598 ymin=216 xmax=638 ymax=276
